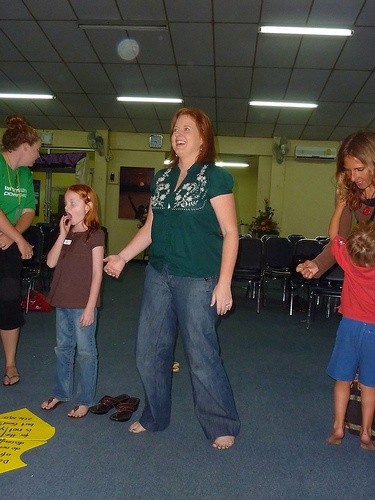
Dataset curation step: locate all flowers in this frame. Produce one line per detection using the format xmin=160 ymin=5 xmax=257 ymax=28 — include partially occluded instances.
xmin=240 ymin=199 xmax=280 ymax=236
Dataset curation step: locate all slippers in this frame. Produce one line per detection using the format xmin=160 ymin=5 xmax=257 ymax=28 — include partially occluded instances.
xmin=41 ymin=396 xmax=60 ymax=411
xmin=3 ymin=370 xmax=20 ymax=386
xmin=67 ymin=404 xmax=88 ymax=418
xmin=110 ymin=397 xmax=140 ymax=422
xmin=88 ymin=393 xmax=130 ymax=415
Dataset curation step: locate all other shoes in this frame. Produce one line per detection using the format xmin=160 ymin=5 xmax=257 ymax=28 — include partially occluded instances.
xmin=171 ymin=361 xmax=180 ymax=373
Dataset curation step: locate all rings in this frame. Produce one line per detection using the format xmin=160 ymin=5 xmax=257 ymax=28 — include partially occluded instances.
xmin=225 ymin=303 xmax=230 ymax=306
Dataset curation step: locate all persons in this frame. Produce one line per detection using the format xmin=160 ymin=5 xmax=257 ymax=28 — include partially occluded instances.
xmin=295 ymin=131 xmax=375 ymax=439
xmin=102 ymin=108 xmax=241 ymax=450
xmin=329 ymin=197 xmax=375 ymax=448
xmin=0 ymin=115 xmax=43 ymax=384
xmin=41 ymin=185 xmax=106 ymax=418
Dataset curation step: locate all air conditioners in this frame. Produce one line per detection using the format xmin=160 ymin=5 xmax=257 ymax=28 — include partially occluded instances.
xmin=295 ymin=146 xmax=337 ymax=160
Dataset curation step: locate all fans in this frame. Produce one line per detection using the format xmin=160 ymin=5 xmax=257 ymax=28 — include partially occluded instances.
xmin=270 ymin=137 xmax=291 ymax=164
xmin=86 ymin=130 xmax=105 ymax=157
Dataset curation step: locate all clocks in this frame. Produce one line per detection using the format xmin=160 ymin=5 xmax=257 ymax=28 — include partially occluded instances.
xmin=39 ymin=131 xmax=52 ymax=145
xmin=149 ymin=134 xmax=163 ymax=148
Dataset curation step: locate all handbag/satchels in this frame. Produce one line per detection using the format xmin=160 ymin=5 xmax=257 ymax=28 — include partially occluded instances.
xmin=342 ymin=369 xmax=375 ymax=441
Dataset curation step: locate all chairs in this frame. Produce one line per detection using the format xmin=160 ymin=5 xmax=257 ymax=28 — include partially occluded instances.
xmin=20 ymin=222 xmax=346 ymax=329
